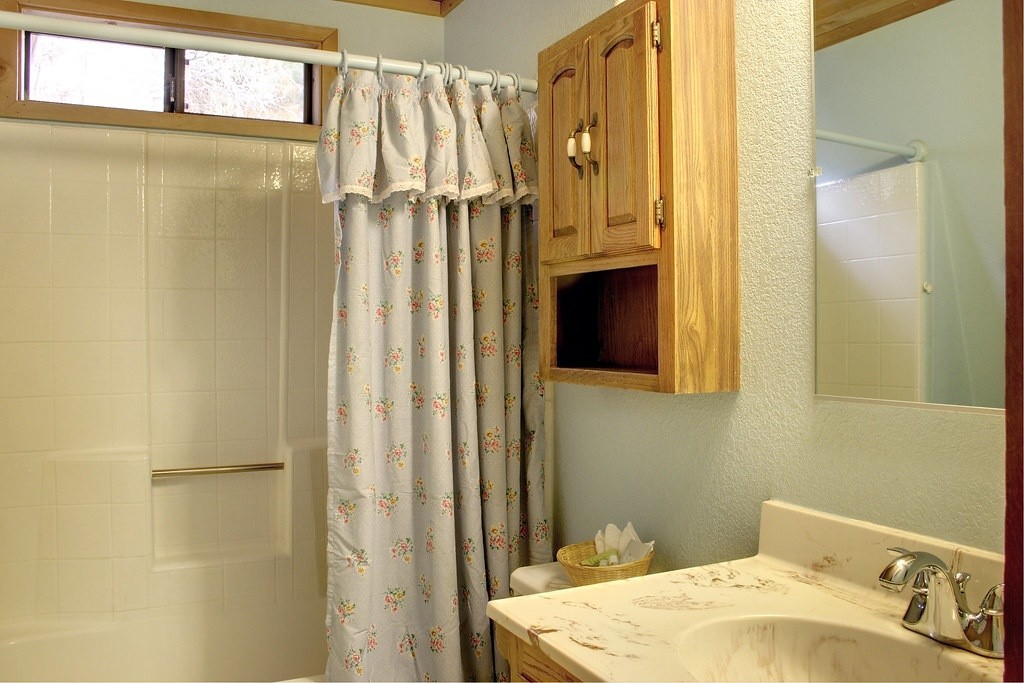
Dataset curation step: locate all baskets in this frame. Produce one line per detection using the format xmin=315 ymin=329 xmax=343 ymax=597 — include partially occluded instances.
xmin=557 ymin=542 xmax=654 ymax=588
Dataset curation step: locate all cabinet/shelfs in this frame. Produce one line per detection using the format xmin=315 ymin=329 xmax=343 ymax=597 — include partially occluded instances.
xmin=538 ymin=1 xmax=741 ymax=395
xmin=486 ymin=626 xmax=583 ymax=683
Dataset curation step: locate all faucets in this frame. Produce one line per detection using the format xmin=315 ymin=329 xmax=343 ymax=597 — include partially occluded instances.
xmin=877 ymin=552 xmax=1004 ymax=658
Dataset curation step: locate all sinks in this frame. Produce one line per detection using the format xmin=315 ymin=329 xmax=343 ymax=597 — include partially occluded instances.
xmin=675 ymin=613 xmax=999 ymax=683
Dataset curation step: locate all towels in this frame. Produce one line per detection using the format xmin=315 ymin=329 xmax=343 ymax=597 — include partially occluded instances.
xmin=595 ymin=521 xmax=655 ymax=565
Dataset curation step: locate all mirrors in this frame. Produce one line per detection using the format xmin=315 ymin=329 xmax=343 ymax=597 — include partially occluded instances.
xmin=815 ymin=1 xmax=1007 ymax=410
